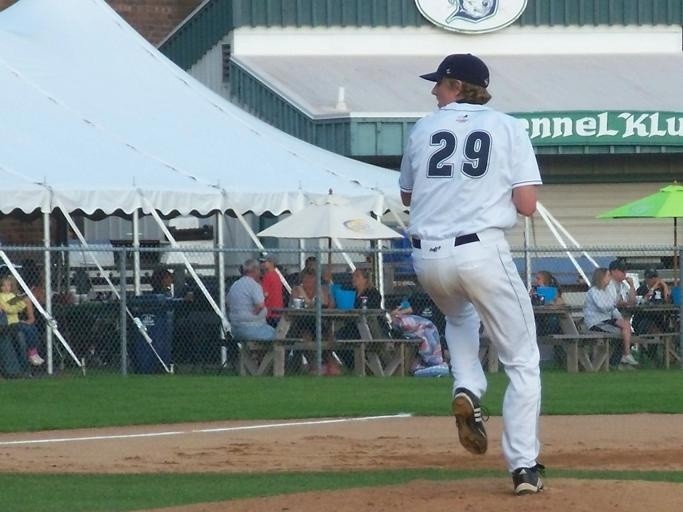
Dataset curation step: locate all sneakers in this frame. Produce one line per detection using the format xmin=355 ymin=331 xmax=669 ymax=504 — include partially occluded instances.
xmin=618 ymin=353 xmax=639 ymax=371
xmin=512 ymin=463 xmax=544 ymax=495
xmin=451 ymin=387 xmax=488 ymax=454
xmin=29 ymin=353 xmax=44 ymax=366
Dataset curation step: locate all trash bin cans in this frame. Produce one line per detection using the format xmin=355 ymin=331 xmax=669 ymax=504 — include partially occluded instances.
xmin=131 ymin=293 xmax=173 ymax=375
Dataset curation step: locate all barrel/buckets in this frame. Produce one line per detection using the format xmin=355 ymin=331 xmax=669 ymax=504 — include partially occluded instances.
xmin=335 ymin=289 xmax=356 ymax=310
xmin=537 ymin=285 xmax=558 ymax=304
xmin=671 ymin=287 xmax=682 ymax=304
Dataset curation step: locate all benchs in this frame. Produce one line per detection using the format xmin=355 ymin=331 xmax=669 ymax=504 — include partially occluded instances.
xmin=483 ymin=300 xmax=682 ymax=373
xmin=242 ymin=304 xmax=420 ymax=376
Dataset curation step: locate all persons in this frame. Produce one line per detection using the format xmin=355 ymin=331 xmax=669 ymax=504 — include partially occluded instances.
xmin=1 ymin=251 xmax=676 ymax=377
xmin=398 ymin=53 xmax=545 ymax=495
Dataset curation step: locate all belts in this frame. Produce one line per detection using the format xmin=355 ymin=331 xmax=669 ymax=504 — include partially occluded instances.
xmin=411 ymin=232 xmax=481 ymax=250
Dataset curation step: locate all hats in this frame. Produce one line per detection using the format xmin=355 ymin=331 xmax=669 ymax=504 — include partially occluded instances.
xmin=258 ymin=252 xmax=273 ymax=262
xmin=644 ymin=268 xmax=658 ymax=278
xmin=0 ymin=261 xmax=23 ymax=269
xmin=609 ymin=260 xmax=625 ymax=272
xmin=419 ymin=53 xmax=489 ymax=88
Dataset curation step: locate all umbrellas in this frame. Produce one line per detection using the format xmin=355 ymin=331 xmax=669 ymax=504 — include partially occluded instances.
xmin=254 ymin=188 xmax=406 ymax=278
xmin=594 ymin=180 xmax=683 ymax=287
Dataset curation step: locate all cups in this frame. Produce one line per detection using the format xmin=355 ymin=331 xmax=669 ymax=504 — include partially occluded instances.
xmin=531 ymin=293 xmax=545 ymax=306
xmin=291 ymin=298 xmax=305 ymax=309
xmin=360 ymin=297 xmax=368 ymax=309
xmin=69 ymin=286 xmax=88 ymax=305
